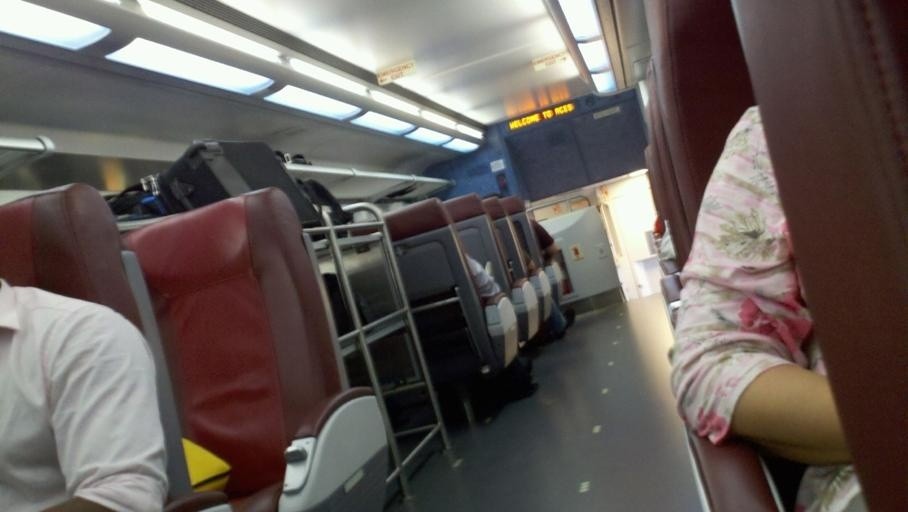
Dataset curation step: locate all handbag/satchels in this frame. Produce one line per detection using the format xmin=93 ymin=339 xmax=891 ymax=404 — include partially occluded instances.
xmin=298 ymin=173 xmax=356 ymax=241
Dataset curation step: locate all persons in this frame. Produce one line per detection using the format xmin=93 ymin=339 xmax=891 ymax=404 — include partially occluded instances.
xmin=532 ymin=218 xmax=560 ymax=260
xmin=666 ymin=102 xmax=871 ymax=511
xmin=457 ymin=241 xmax=540 ymax=405
xmin=0 ymin=275 xmax=170 ymax=512
xmin=523 ymin=251 xmax=577 ymax=340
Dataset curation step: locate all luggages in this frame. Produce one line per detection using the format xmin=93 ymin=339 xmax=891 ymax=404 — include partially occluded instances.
xmin=146 ymin=128 xmax=330 ymax=245
